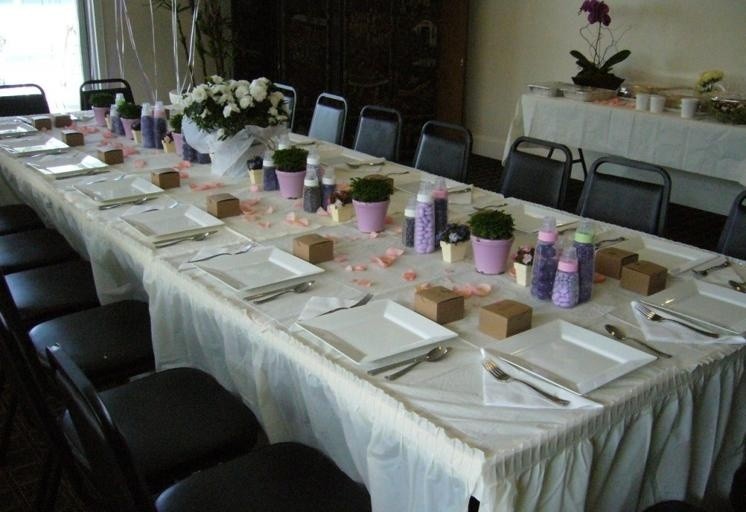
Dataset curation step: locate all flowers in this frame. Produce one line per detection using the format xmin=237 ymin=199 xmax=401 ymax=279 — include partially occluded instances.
xmin=569 ymin=1 xmax=631 ymax=87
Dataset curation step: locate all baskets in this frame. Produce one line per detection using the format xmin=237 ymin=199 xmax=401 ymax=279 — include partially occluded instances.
xmin=529 ymin=83 xmax=617 ymax=101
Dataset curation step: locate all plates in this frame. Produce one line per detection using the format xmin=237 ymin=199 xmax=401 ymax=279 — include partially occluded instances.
xmin=482 ymin=318 xmax=658 ymax=400
xmin=281 ymin=125 xmax=746 ymax=292
xmin=644 ymin=276 xmax=746 ymax=336
xmin=33 ymin=109 xmax=95 ymax=123
xmin=0 ymin=116 xmax=38 ymax=138
xmin=118 ymin=203 xmax=226 ymax=239
xmin=71 ymin=173 xmax=164 ymax=204
xmin=2 ymin=134 xmax=70 ymax=156
xmin=20 ymin=149 xmax=110 ymax=178
xmin=296 ymin=297 xmax=458 ymax=366
xmin=190 ymin=245 xmax=326 ymax=294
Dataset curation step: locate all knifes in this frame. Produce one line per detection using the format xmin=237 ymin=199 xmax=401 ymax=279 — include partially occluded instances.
xmin=245 ymin=280 xmax=315 ymax=300
xmin=152 ymin=229 xmax=217 ymax=244
xmin=366 ymin=346 xmax=454 ymax=376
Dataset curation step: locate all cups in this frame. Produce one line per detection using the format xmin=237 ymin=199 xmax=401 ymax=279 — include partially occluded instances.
xmin=636 ymin=94 xmax=650 ymax=110
xmin=681 ymin=99 xmax=700 ymax=119
xmin=649 ymin=96 xmax=666 ymax=113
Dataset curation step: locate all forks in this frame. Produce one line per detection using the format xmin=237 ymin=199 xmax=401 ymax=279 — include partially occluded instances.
xmin=557 ymin=226 xmax=576 ymax=235
xmin=386 ymin=170 xmax=410 ymax=177
xmin=596 ymin=236 xmax=627 ymax=248
xmin=473 ymin=203 xmax=508 ymax=211
xmin=55 ymin=170 xmax=109 ymax=180
xmin=634 ymin=300 xmax=721 ymax=339
xmin=319 ymin=292 xmax=375 ymax=316
xmin=447 ymin=188 xmax=471 ymax=194
xmin=691 ymin=256 xmax=733 ymax=278
xmin=98 ymin=196 xmax=156 ymax=210
xmin=481 ymin=358 xmax=570 ymax=407
xmin=345 ymin=161 xmax=384 ymax=168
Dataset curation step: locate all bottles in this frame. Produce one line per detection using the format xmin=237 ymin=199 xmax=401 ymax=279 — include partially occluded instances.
xmin=109 ymin=93 xmax=596 ymax=308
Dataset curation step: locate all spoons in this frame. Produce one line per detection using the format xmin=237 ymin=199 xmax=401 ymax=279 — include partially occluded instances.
xmin=602 ymin=322 xmax=673 ymax=359
xmin=254 ymin=280 xmax=313 ymax=305
xmin=385 ymin=345 xmax=447 ymax=381
xmin=155 ymin=232 xmax=209 ymax=249
xmin=728 ymin=281 xmax=746 ymax=293
